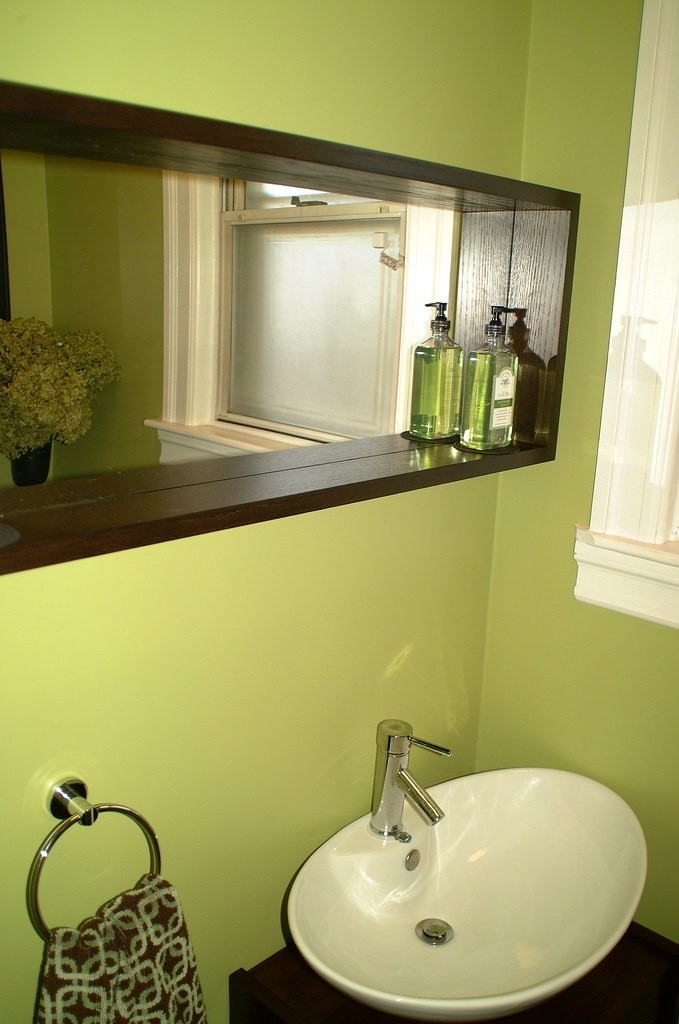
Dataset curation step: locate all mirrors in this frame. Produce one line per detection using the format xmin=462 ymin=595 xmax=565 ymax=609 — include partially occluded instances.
xmin=0 ymin=149 xmax=514 ymax=517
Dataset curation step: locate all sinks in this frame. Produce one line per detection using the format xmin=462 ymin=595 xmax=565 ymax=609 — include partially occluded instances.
xmin=288 ymin=767 xmax=648 ymax=1019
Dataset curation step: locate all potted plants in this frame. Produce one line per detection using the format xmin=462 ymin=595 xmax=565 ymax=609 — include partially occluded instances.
xmin=0 ymin=317 xmax=122 ymax=488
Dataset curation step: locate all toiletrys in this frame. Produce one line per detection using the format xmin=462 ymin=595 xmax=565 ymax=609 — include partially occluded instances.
xmin=459 ymin=306 xmax=522 ymax=451
xmin=410 ymin=300 xmax=463 ymax=440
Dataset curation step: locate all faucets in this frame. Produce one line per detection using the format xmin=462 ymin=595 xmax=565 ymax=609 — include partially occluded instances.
xmin=371 ymin=718 xmax=454 ymax=838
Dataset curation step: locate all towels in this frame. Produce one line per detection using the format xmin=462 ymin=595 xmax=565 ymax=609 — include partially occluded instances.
xmin=32 ymin=874 xmax=208 ymax=1024
